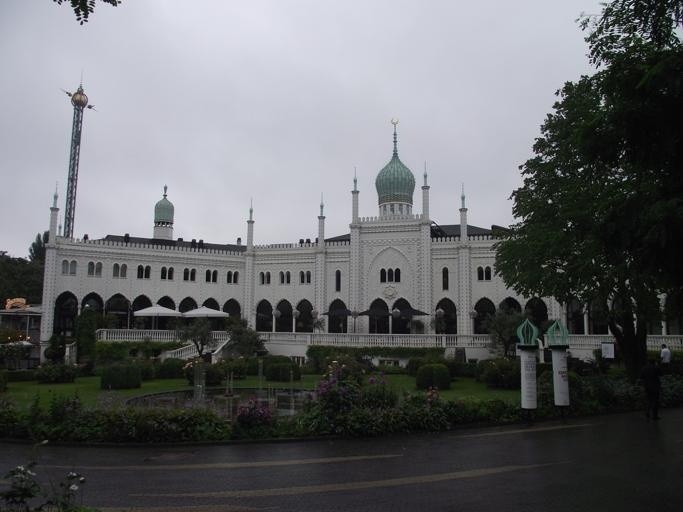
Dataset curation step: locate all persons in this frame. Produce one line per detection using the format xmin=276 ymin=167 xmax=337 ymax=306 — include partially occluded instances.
xmin=639 ymin=357 xmax=665 ymax=420
xmin=660 ymin=344 xmax=672 ymax=369
